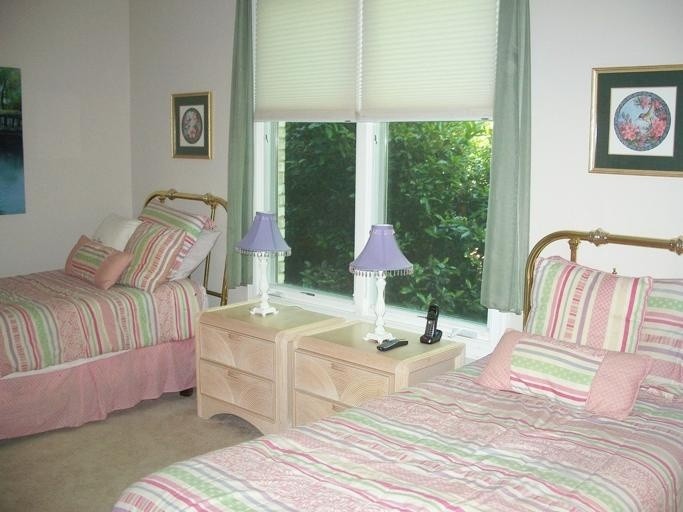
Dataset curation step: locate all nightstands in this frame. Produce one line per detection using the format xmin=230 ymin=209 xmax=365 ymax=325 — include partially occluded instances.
xmin=195 ymin=299 xmax=345 ymax=434
xmin=292 ymin=320 xmax=465 ymax=428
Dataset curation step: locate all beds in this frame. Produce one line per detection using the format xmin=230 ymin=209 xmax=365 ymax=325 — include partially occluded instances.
xmin=110 ymin=230 xmax=683 ymax=510
xmin=0 ymin=189 xmax=228 ymax=439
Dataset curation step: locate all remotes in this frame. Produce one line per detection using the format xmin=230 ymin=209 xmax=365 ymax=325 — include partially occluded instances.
xmin=376 ymin=338 xmax=409 ymax=352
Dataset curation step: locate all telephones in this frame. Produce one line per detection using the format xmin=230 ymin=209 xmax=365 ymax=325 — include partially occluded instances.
xmin=420 ymin=303 xmax=443 ymax=344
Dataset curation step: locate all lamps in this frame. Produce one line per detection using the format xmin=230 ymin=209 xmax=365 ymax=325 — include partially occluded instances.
xmin=235 ymin=212 xmax=290 ymax=316
xmin=349 ymin=224 xmax=414 ymax=342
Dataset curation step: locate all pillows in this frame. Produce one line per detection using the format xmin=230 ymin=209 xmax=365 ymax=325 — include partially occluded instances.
xmin=64 ymin=235 xmax=131 ymax=289
xmin=91 ymin=208 xmax=144 ymax=251
xmin=472 ymin=328 xmax=654 ymax=417
xmin=636 ymin=277 xmax=683 ymax=399
xmin=175 ymin=226 xmax=221 ymax=281
xmin=524 ymin=256 xmax=652 ymax=354
xmin=139 ymin=198 xmax=208 ymax=282
xmin=117 ymin=222 xmax=187 ymax=292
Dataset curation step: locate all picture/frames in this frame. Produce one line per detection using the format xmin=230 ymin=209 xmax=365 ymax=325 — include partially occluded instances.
xmin=171 ymin=92 xmax=213 ymax=160
xmin=588 ymin=64 xmax=683 ymax=176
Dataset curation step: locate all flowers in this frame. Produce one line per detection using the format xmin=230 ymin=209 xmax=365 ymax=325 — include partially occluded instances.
xmin=617 ymin=95 xmax=667 ymax=148
